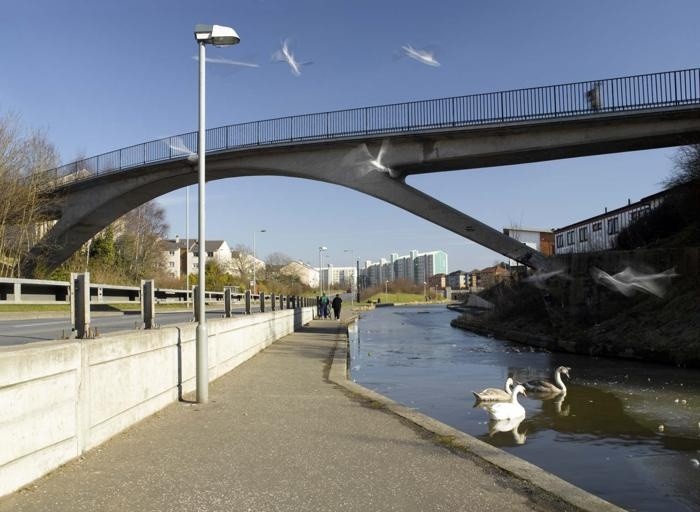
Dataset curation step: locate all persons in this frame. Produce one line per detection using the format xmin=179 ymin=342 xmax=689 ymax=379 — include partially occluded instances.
xmin=331 ymin=294 xmax=343 ymax=321
xmin=586 ymin=82 xmax=602 ymax=113
xmin=319 ymin=291 xmax=330 ymax=320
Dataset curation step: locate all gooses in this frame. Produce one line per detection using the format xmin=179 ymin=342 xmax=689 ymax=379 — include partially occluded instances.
xmin=480 ymin=383 xmax=528 ymax=421
xmin=530 ymin=391 xmax=572 ymax=418
xmin=486 ymin=419 xmax=529 ymax=446
xmin=515 ymin=364 xmax=570 ymax=391
xmin=472 ymin=375 xmax=514 ymax=401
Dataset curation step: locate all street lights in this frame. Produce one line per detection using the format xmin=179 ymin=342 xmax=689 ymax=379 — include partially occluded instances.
xmin=253 ymin=230 xmax=266 ymax=294
xmin=386 ymin=280 xmax=389 ymax=294
xmin=319 ymin=246 xmax=328 ymax=296
xmin=344 ymin=250 xmax=353 ymax=306
xmin=194 ymin=24 xmax=240 ymax=403
xmin=424 ymin=282 xmax=426 ymax=295
